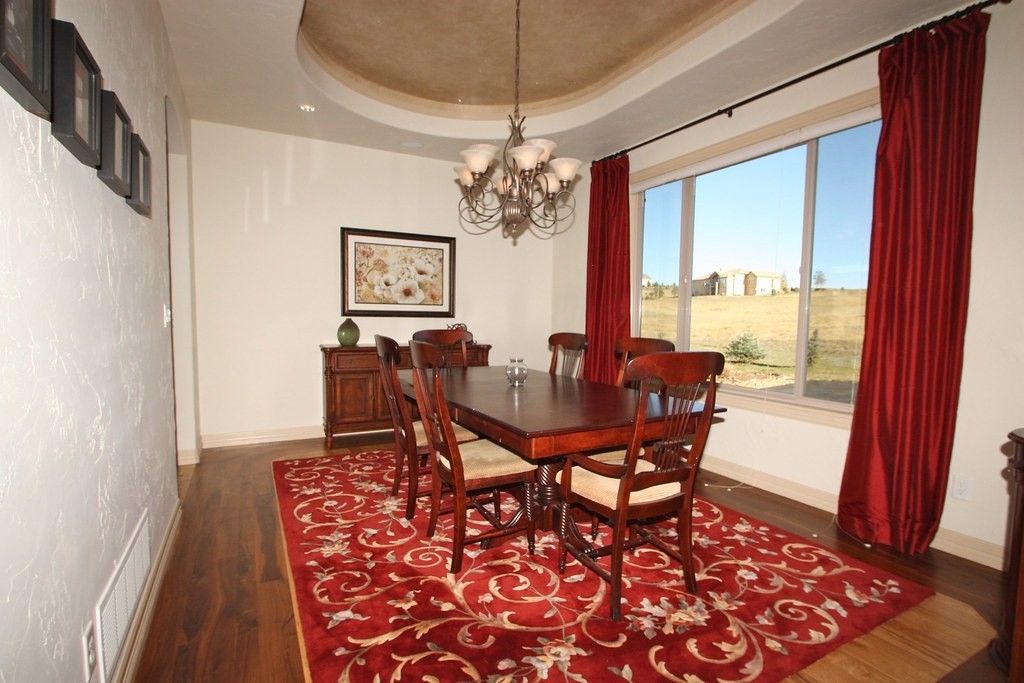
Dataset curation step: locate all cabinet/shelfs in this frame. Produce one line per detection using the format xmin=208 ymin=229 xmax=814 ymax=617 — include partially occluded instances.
xmin=317 ymin=343 xmax=492 ymax=448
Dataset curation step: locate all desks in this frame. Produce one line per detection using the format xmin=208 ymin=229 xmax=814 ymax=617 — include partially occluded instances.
xmin=388 ymin=366 xmax=728 ymax=548
xmin=989 ymin=422 xmax=1024 ymax=683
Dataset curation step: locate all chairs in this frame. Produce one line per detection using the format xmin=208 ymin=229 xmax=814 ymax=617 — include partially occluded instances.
xmin=555 ymin=351 xmax=728 ymax=621
xmin=548 ymin=333 xmax=590 ymax=381
xmin=373 ymin=334 xmax=479 ymax=520
xmin=607 ymin=337 xmax=676 ymax=394
xmin=405 ymin=340 xmax=540 ymax=576
xmin=412 ymin=329 xmax=473 ymax=366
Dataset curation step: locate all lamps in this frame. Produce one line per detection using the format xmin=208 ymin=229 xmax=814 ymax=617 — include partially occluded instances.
xmin=452 ymin=1 xmax=584 ymax=236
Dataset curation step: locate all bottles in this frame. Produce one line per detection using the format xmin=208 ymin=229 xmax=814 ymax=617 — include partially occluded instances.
xmin=505 ymin=358 xmax=527 ymax=386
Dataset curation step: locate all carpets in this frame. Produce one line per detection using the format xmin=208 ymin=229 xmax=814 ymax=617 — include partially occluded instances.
xmin=272 ymin=451 xmax=938 ymax=683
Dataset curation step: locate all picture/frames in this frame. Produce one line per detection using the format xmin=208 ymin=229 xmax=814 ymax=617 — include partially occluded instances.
xmin=51 ymin=19 xmax=101 ymax=167
xmin=0 ymin=0 xmax=51 ymax=119
xmin=97 ymin=89 xmax=131 ymax=197
xmin=340 ymin=227 xmax=456 ymax=318
xmin=126 ymin=133 xmax=151 ymax=217
